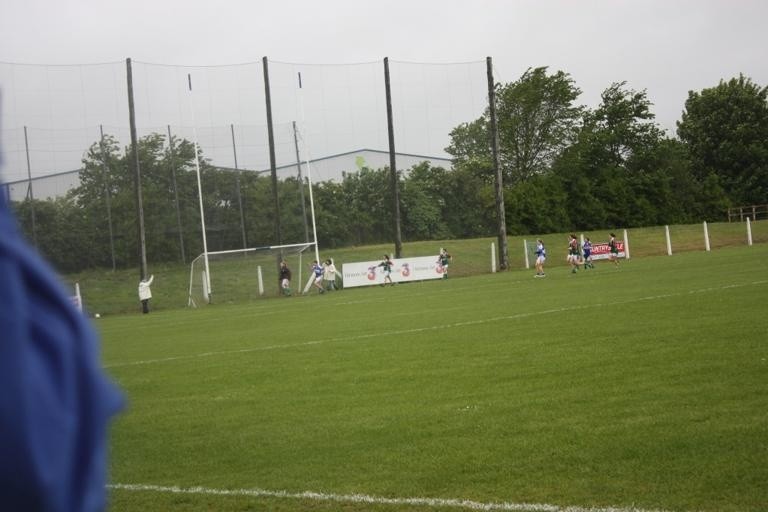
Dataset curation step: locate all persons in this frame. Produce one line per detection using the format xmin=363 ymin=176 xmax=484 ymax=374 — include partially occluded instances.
xmin=323 ymin=259 xmax=338 ymax=291
xmin=437 ymin=249 xmax=453 ymax=281
xmin=608 ymin=233 xmax=620 ymax=266
xmin=279 ymin=262 xmax=293 ymax=297
xmin=138 ymin=275 xmax=154 ymax=313
xmin=566 ymin=234 xmax=595 ymax=273
xmin=376 ymin=254 xmax=394 ymax=287
xmin=534 ymin=239 xmax=546 ymax=278
xmin=0 ymin=159 xmax=130 ymax=512
xmin=307 ymin=261 xmax=326 ymax=293
